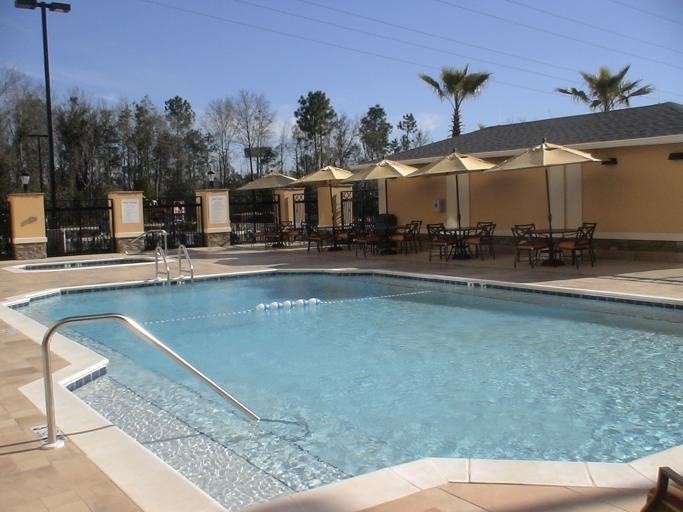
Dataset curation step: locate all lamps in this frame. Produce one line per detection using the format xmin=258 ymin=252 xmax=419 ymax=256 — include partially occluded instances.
xmin=206 ymin=169 xmax=215 ymax=189
xmin=18 ymin=171 xmax=29 ymax=192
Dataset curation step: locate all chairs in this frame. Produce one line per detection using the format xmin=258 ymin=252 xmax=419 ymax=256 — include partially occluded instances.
xmin=247 ymin=220 xmax=496 ymax=261
xmin=509 ymin=222 xmax=599 ymax=270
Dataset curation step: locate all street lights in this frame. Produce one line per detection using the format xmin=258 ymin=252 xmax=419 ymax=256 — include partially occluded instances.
xmin=205 ymin=166 xmax=216 ymax=188
xmin=11 ymin=0 xmax=70 ymax=230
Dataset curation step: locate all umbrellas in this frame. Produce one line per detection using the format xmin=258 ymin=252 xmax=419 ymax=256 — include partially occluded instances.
xmin=405 ymin=147 xmax=498 ymax=253
xmin=282 ymin=161 xmax=353 ymax=245
xmin=482 ymin=136 xmax=604 ymax=259
xmin=233 ymin=170 xmax=298 ymax=241
xmin=340 ymin=155 xmax=418 ymax=249
